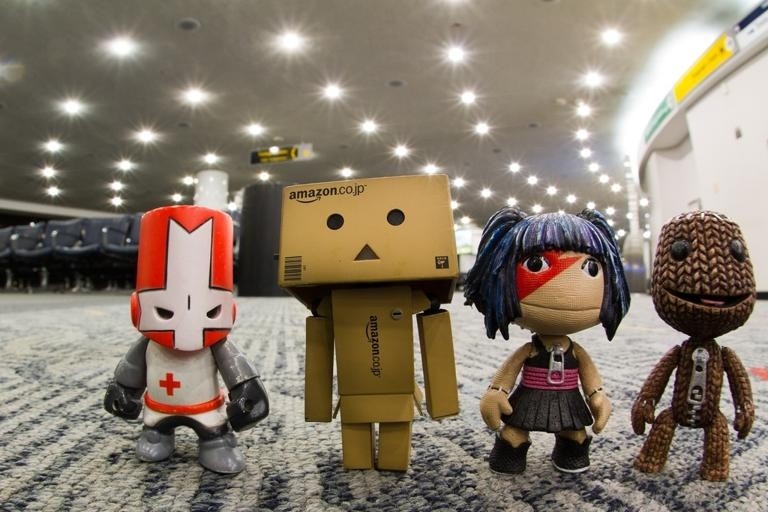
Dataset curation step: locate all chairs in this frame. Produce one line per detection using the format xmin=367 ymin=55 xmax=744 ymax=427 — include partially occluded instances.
xmin=0 ymin=210 xmax=172 ymax=294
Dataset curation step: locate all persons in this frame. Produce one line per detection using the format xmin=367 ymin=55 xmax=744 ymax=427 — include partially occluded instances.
xmin=463 ymin=205 xmax=630 ymax=475
